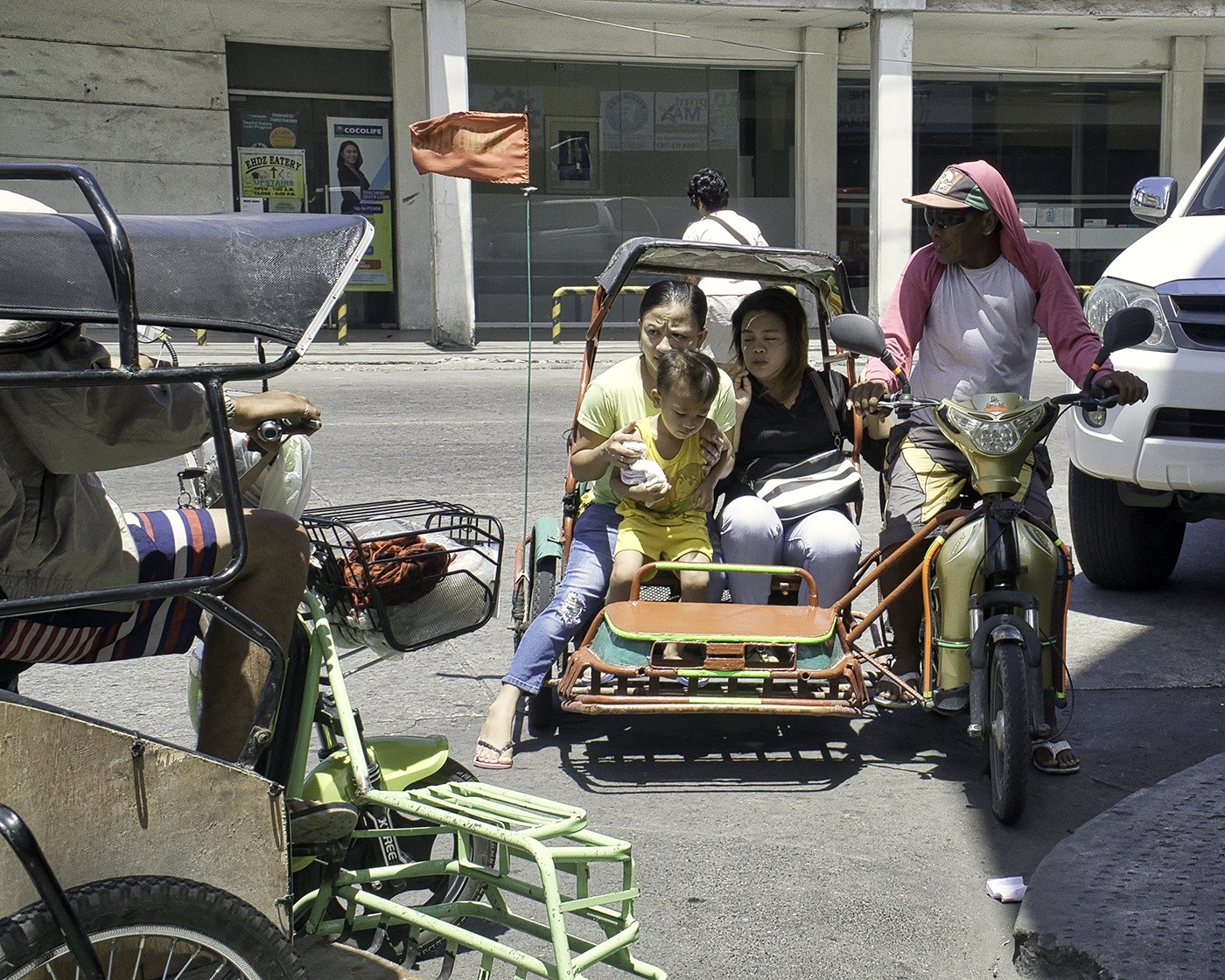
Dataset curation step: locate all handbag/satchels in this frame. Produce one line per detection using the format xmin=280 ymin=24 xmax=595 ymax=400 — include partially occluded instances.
xmin=748 ymin=449 xmax=864 ymax=525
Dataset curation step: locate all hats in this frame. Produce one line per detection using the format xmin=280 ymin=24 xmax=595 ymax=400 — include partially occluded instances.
xmin=902 ymin=168 xmax=993 ymax=212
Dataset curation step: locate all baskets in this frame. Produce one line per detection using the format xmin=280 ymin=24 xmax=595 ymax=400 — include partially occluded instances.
xmin=299 ymin=500 xmax=504 ymax=652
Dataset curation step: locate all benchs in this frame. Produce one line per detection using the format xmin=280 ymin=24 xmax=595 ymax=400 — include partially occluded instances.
xmin=564 ymin=366 xmax=865 ymax=591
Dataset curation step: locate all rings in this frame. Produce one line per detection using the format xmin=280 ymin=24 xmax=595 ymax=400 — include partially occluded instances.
xmin=302 ymin=404 xmax=311 ymax=419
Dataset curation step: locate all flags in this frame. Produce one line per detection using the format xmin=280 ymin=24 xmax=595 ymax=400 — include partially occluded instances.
xmin=409 ymin=106 xmax=532 ymax=184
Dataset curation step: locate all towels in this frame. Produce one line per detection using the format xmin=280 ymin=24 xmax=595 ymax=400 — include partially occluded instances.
xmin=620 ymin=429 xmax=670 ymax=509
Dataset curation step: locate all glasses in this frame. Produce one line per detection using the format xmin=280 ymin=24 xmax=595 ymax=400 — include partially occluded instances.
xmin=923 ymin=206 xmax=978 ymax=229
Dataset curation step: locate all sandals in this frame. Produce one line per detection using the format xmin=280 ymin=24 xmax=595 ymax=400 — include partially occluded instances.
xmin=745 ymin=646 xmax=783 ymax=667
xmin=288 ymin=800 xmax=358 ymax=843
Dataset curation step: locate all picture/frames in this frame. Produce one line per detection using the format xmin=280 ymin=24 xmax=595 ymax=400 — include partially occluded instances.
xmin=544 ymin=116 xmax=605 ymax=196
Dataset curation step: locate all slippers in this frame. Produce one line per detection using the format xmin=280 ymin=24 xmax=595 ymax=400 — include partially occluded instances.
xmin=581 ymin=666 xmax=615 ymax=686
xmin=473 ymin=736 xmax=516 ymax=768
xmin=676 ymin=676 xmax=710 ymax=688
xmin=1032 ymin=739 xmax=1080 ymax=774
xmin=873 ymin=672 xmax=920 ymax=709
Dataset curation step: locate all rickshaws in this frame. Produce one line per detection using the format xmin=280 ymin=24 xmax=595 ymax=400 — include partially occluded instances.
xmin=508 ymin=235 xmax=1154 ymax=824
xmin=0 ymin=165 xmax=667 ymax=980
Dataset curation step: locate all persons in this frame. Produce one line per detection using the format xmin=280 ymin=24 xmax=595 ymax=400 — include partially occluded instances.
xmin=0 ymin=317 xmax=323 ymax=767
xmin=845 ymin=159 xmax=1149 ymax=709
xmin=472 ymin=280 xmax=892 ymax=770
xmin=603 ymin=347 xmax=733 ymax=660
xmin=681 ymin=165 xmax=784 ymax=379
xmin=337 ymin=140 xmax=370 ymax=215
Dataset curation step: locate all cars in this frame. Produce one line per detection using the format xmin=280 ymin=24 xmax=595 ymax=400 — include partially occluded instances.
xmin=472 ymin=197 xmax=662 ymax=259
xmin=1068 ymin=135 xmax=1225 ymax=596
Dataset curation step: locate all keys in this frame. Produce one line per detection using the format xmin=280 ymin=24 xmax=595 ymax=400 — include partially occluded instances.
xmin=177 ymin=467 xmax=207 ymax=481
